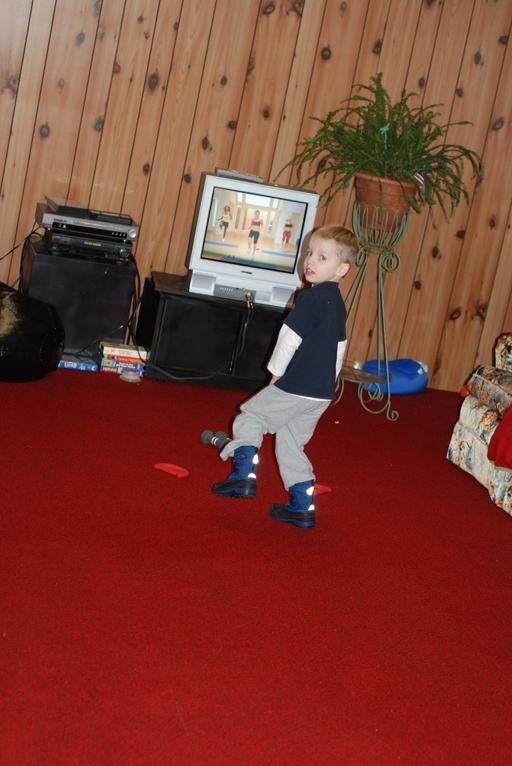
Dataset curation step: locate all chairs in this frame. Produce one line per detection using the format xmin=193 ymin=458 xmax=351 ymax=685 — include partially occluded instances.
xmin=444 ymin=332 xmax=512 ymax=517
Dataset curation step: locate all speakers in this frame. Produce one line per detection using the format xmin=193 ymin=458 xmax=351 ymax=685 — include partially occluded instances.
xmin=17 ymin=236 xmax=138 ymax=355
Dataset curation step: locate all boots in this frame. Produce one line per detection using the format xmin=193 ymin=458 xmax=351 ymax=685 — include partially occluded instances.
xmin=212 ymin=446 xmax=258 ymax=499
xmin=270 ymin=480 xmax=315 ymax=529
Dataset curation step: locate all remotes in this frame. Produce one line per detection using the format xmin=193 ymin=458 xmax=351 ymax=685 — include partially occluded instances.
xmin=214 ymin=167 xmax=266 ymax=184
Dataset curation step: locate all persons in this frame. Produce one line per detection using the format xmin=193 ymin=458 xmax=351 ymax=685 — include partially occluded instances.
xmin=208 ymin=223 xmax=361 ymax=531
xmin=216 ymin=205 xmax=233 ymax=242
xmin=279 ymin=217 xmax=295 ymax=251
xmin=245 ymin=210 xmax=264 ymax=259
xmin=266 ymin=221 xmax=274 ymax=233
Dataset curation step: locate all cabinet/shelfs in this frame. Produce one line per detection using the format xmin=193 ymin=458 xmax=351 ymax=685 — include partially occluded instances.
xmin=133 ymin=268 xmax=298 ymax=392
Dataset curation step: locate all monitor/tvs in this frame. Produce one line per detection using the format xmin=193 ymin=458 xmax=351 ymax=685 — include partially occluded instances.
xmin=184 ymin=171 xmax=321 ymax=309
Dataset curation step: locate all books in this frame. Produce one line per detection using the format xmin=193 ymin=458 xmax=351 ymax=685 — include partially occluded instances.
xmin=97 ymin=364 xmax=142 ymax=378
xmin=93 ymin=354 xmax=141 ymax=371
xmin=98 ymin=351 xmax=142 ymax=364
xmin=56 ymin=352 xmax=99 ymax=372
xmin=97 ymin=341 xmax=147 ymax=360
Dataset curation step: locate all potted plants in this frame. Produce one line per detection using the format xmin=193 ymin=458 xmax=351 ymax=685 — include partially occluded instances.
xmin=270 ymin=69 xmax=487 ymax=236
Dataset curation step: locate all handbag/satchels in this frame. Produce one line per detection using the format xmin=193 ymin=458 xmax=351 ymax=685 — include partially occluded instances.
xmin=364 ymin=359 xmax=428 ymax=395
